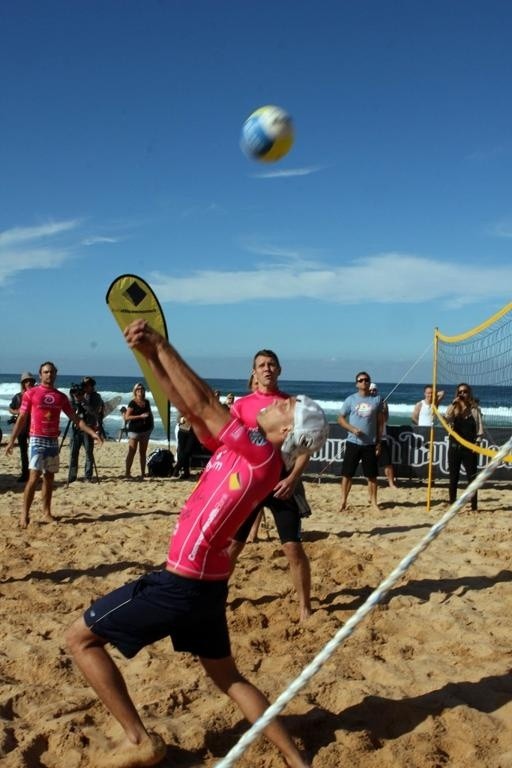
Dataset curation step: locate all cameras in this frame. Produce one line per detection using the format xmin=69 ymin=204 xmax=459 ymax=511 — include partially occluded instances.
xmin=6 ymin=415 xmax=18 ymax=425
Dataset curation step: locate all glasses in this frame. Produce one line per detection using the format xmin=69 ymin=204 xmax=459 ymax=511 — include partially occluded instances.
xmin=458 ymin=390 xmax=468 ymax=394
xmin=356 ymin=378 xmax=370 ymax=382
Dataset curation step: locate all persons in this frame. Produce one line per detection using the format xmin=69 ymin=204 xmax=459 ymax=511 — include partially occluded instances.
xmin=64 ymin=318 xmax=328 ymax=768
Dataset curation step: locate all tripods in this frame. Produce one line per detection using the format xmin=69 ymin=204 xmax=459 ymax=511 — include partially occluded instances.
xmin=57 ymin=406 xmax=101 ymax=489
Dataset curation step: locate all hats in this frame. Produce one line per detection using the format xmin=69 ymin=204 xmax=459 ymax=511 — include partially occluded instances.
xmin=369 ymin=383 xmax=378 ymax=391
xmin=20 ymin=371 xmax=36 ymax=384
xmin=281 ymin=394 xmax=329 ymax=472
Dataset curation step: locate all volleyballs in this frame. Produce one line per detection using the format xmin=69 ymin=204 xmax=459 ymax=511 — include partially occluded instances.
xmin=244 ymin=104 xmax=294 ymax=162
